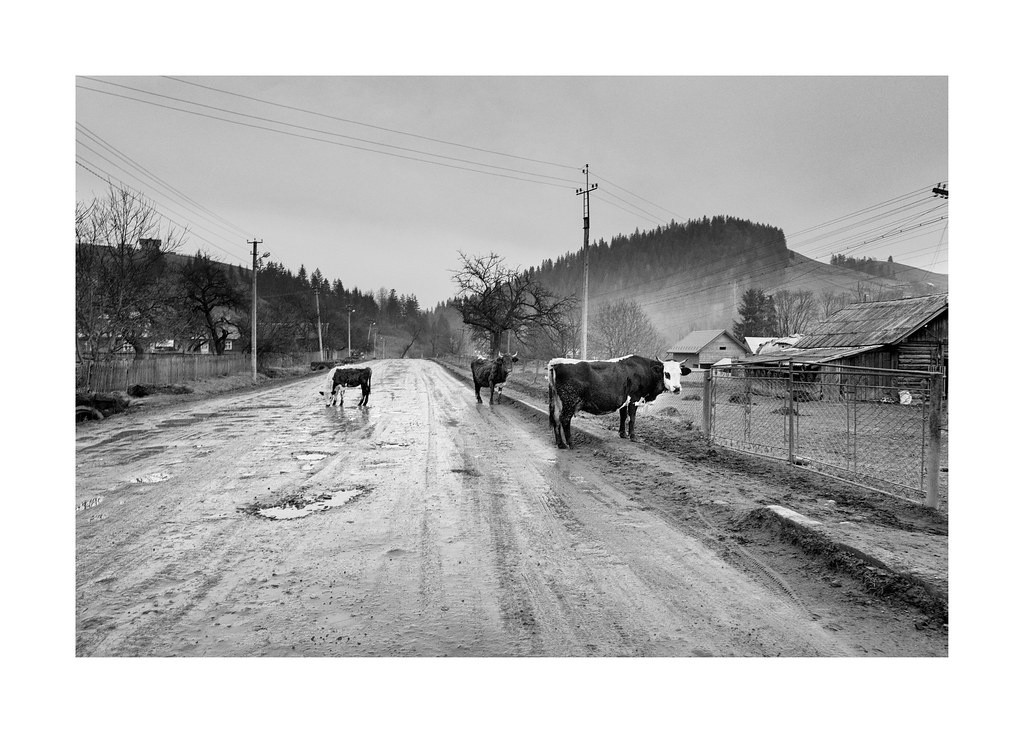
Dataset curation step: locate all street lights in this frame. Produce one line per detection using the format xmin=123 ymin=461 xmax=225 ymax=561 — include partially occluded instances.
xmin=349 ymin=310 xmax=355 ymax=357
xmin=365 ymin=322 xmax=375 ymax=358
xmin=252 ymin=251 xmax=270 ymax=380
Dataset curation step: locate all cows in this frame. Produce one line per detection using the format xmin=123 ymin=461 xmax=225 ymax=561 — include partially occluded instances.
xmin=547 ymin=354 xmax=692 ymax=449
xmin=319 ymin=366 xmax=372 ymax=407
xmin=471 ymin=350 xmax=519 ymax=405
